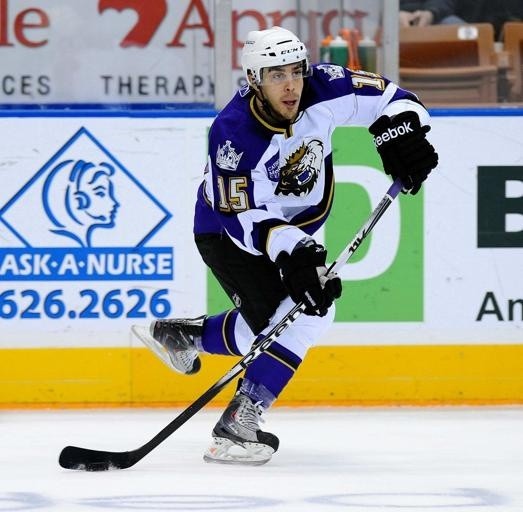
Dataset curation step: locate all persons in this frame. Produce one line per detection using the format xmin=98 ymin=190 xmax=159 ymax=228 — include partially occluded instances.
xmin=398 ymin=0 xmax=465 ymax=27
xmin=147 ymin=24 xmax=439 ymax=456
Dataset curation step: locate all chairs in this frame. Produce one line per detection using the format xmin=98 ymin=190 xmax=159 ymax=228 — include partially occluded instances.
xmin=399 ymin=21 xmax=523 ymax=107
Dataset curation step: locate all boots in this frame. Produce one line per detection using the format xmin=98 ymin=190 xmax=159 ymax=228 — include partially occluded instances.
xmin=150 ymin=314 xmax=209 ymax=374
xmin=212 ymin=376 xmax=280 ymax=452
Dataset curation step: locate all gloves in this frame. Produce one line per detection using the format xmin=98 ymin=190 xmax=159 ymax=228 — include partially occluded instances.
xmin=274 ymin=236 xmax=342 ymax=319
xmin=367 ymin=109 xmax=438 ymax=196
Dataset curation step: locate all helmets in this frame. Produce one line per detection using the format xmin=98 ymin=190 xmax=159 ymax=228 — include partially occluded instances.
xmin=242 ymin=24 xmax=315 ymax=88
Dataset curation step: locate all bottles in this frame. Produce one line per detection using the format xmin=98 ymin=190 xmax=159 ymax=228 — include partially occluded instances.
xmin=316 ymin=28 xmax=376 ymax=75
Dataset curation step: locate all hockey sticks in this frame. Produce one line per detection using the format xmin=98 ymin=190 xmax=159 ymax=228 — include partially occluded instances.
xmin=58 ymin=179 xmax=402 ymax=472
